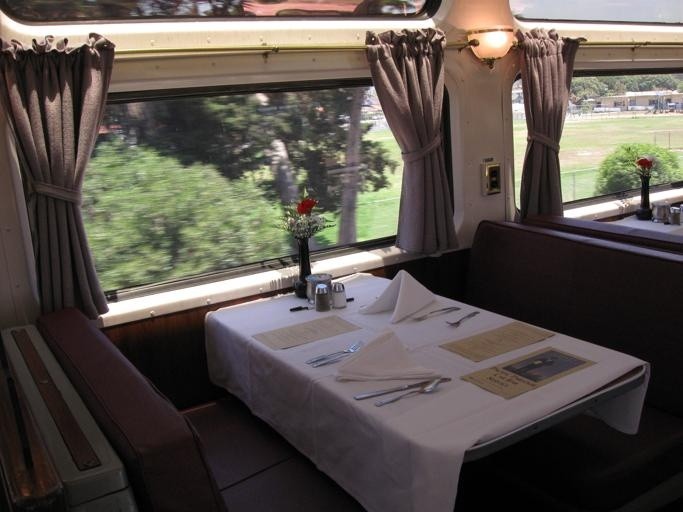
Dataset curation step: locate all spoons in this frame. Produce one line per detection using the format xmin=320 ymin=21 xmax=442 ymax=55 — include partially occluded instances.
xmin=373 ymin=375 xmax=443 ymax=410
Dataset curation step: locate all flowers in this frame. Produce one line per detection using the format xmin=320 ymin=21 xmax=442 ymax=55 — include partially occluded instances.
xmin=616 ymin=143 xmax=655 ymax=180
xmin=271 ymin=193 xmax=336 ymax=239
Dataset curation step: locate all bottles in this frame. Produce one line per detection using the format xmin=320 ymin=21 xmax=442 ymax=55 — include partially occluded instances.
xmin=314 ymin=284 xmax=330 ymax=313
xmin=332 ymin=283 xmax=347 ymax=309
xmin=650 ymin=200 xmax=682 ymax=225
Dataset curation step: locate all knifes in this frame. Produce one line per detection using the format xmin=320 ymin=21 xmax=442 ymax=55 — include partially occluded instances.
xmin=334 ymin=375 xmax=451 ymax=385
xmin=353 ymin=375 xmax=450 ymax=399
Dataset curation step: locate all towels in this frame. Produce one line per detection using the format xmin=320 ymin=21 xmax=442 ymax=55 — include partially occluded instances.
xmin=356 ymin=266 xmax=435 ymax=324
xmin=330 ymin=330 xmax=438 ymax=383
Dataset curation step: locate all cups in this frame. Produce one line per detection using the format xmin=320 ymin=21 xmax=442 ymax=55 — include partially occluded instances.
xmin=304 ymin=273 xmax=331 ymax=304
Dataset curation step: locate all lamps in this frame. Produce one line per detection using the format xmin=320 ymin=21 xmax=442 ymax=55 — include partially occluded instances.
xmin=460 ymin=26 xmax=517 ymax=70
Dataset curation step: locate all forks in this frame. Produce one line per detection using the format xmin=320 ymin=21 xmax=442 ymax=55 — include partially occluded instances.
xmin=408 ymin=306 xmax=459 ymax=321
xmin=305 ymin=344 xmax=361 ymax=368
xmin=447 ymin=310 xmax=480 ymax=328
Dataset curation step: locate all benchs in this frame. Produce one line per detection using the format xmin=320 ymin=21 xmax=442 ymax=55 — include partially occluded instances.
xmin=34 ymin=305 xmax=360 ymax=512
xmin=0 ymin=340 xmax=65 ymax=511
xmin=523 ymin=214 xmax=682 ymax=252
xmin=445 ymin=215 xmax=683 ymax=512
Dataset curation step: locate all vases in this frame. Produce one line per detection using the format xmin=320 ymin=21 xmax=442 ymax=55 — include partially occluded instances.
xmin=635 ymin=175 xmax=655 ymax=221
xmin=294 ymin=236 xmax=314 ymax=299
